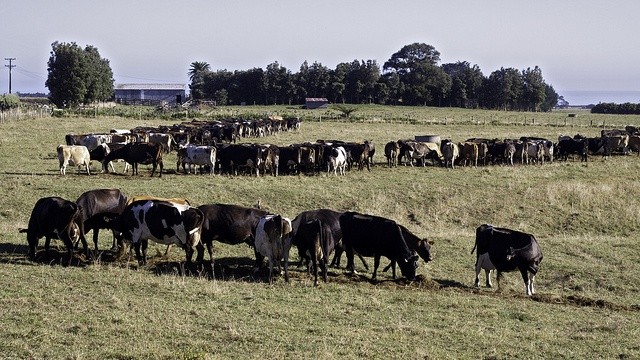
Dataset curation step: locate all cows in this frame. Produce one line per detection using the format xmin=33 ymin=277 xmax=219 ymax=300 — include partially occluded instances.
xmin=71 ymin=189 xmax=127 ymax=256
xmin=291 ymin=210 xmax=343 ymax=268
xmin=255 ymin=215 xmax=292 ymax=285
xmin=116 ymin=196 xmax=205 ymax=256
xmin=337 ymin=224 xmax=435 ymax=279
xmin=57 ymin=117 xmax=375 ymax=177
xmin=296 ymin=218 xmax=335 ymax=287
xmin=339 ymin=211 xmax=419 ymax=282
xmin=196 ymin=204 xmax=274 ymax=280
xmin=385 ymin=126 xmax=640 ymax=169
xmin=471 ymin=224 xmax=543 ymax=295
xmin=18 ymin=197 xmax=80 ymax=267
xmin=98 ymin=200 xmax=204 ymax=266
